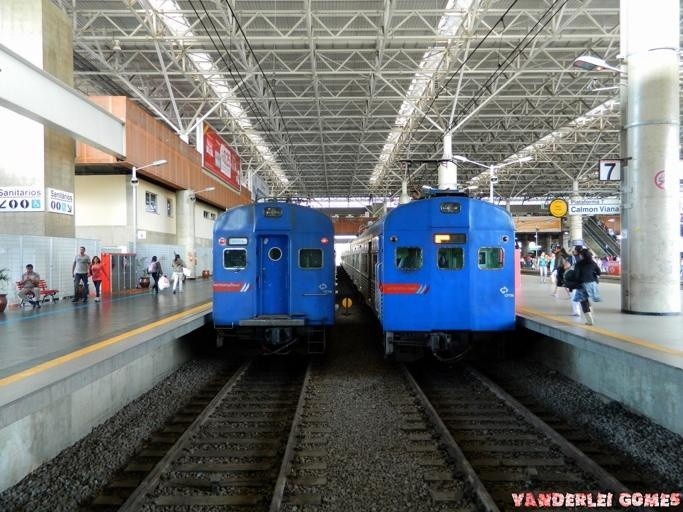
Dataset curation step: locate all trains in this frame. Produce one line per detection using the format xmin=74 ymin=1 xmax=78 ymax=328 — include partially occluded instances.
xmin=340 ymin=188 xmax=515 ymax=363
xmin=212 ymin=202 xmax=335 ymax=354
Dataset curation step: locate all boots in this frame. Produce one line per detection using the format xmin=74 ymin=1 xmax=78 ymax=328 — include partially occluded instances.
xmin=583 ymin=305 xmax=593 ymax=325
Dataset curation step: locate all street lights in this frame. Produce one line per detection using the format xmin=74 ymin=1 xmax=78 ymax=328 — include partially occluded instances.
xmin=130 ymin=159 xmax=168 ymax=253
xmin=188 ymin=186 xmax=216 ymax=249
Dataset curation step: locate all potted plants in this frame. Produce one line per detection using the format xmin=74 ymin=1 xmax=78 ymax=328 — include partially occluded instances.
xmin=139 ymin=267 xmax=151 ymax=288
xmin=0 ymin=267 xmax=14 ymax=312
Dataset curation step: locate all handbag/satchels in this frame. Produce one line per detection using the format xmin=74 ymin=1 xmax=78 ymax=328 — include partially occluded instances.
xmin=158 ymin=276 xmax=170 ymax=290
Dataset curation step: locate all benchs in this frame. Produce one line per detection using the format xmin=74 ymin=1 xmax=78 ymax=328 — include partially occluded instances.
xmin=16 ymin=280 xmax=59 ymax=307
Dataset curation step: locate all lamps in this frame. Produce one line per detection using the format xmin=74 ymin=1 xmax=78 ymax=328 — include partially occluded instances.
xmin=572 ymin=48 xmax=630 ymax=77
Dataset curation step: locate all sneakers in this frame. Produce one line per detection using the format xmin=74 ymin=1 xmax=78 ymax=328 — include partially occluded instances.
xmin=31 ymin=301 xmax=40 ymax=308
xmin=152 ymin=282 xmax=156 ymax=289
xmin=94 ymin=297 xmax=100 ymax=302
xmin=71 ymin=298 xmax=88 ymax=302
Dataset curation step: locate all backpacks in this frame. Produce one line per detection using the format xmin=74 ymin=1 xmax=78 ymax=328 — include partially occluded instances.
xmin=147 ymin=261 xmax=160 ymax=274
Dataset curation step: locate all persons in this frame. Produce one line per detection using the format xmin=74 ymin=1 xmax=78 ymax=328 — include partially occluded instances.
xmin=520 ymin=245 xmax=601 ymax=326
xmin=71 ymin=246 xmax=108 ymax=302
xmin=18 ymin=264 xmax=41 ymax=308
xmin=152 ymin=294 xmax=182 ymax=316
xmin=74 ymin=303 xmax=103 ymax=344
xmin=147 ymin=255 xmax=187 ymax=294
